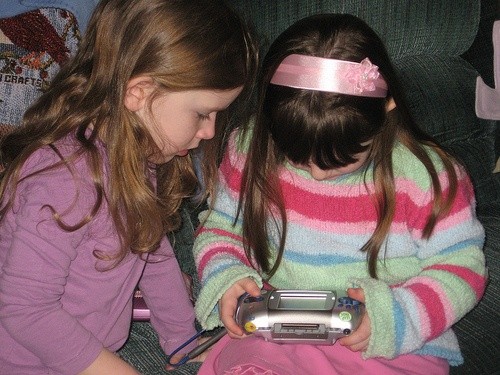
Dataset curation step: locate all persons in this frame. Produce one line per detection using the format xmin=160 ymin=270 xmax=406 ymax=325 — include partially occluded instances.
xmin=0 ymin=0 xmax=259 ymax=375
xmin=192 ymin=12 xmax=489 ymax=375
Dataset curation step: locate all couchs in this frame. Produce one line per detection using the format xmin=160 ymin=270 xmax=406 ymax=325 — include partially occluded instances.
xmin=118 ymin=0 xmax=500 ymax=375
